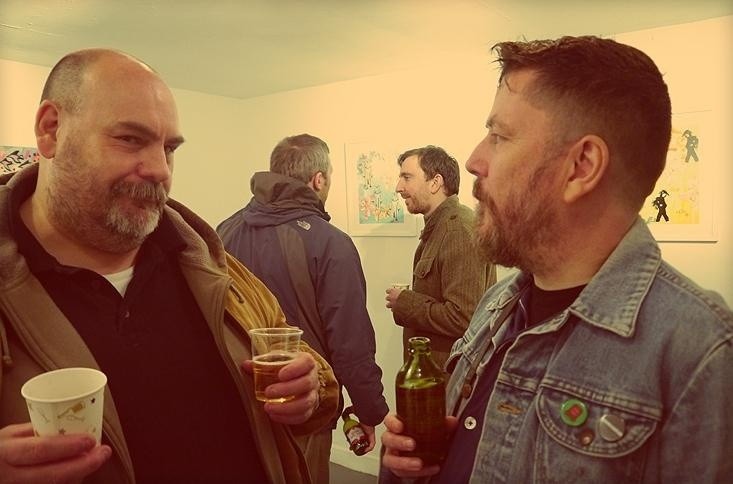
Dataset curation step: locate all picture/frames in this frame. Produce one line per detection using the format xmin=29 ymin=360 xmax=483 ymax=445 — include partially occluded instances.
xmin=343 ymin=140 xmax=420 ymax=238
xmin=638 ymin=104 xmax=720 ymax=242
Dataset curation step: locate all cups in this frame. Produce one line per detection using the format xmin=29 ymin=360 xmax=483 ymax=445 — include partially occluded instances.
xmin=248 ymin=327 xmax=305 ymax=404
xmin=392 ymin=284 xmax=411 ymax=292
xmin=21 ymin=367 xmax=109 ymax=450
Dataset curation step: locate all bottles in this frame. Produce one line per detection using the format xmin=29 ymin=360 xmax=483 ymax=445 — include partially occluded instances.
xmin=394 ymin=337 xmax=449 ymax=466
xmin=341 ymin=415 xmax=369 ymax=457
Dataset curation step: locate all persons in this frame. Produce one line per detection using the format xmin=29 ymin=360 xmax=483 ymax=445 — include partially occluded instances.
xmin=0 ymin=47 xmax=342 ymax=483
xmin=387 ymin=146 xmax=496 ymax=367
xmin=381 ymin=36 xmax=732 ymax=484
xmin=215 ymin=134 xmax=389 ymax=454
